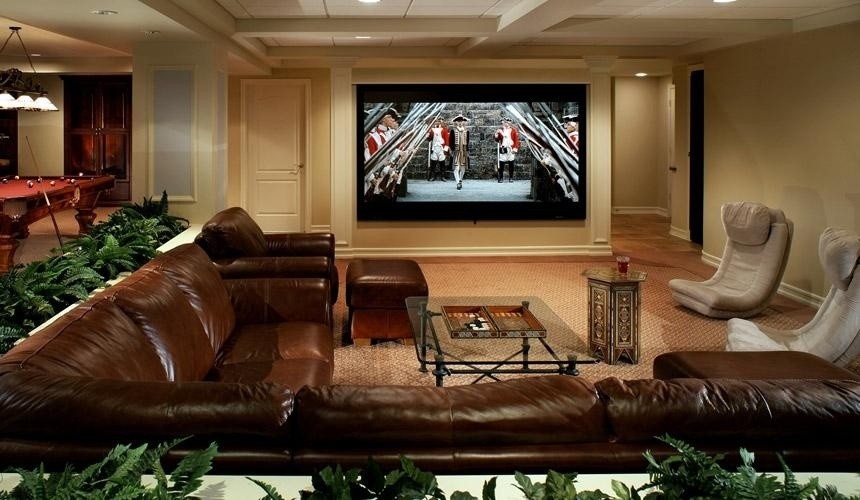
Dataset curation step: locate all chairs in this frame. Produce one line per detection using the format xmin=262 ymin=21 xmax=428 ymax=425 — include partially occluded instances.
xmin=724 ymin=226 xmax=860 ymax=369
xmin=193 ymin=207 xmax=339 ymax=328
xmin=666 ymin=201 xmax=794 ymax=320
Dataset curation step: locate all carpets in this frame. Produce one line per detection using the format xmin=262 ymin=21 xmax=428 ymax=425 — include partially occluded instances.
xmin=330 ymin=263 xmax=806 ymax=387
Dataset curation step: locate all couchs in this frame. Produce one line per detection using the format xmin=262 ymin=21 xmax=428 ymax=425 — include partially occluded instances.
xmin=0 ymin=243 xmax=860 ymax=474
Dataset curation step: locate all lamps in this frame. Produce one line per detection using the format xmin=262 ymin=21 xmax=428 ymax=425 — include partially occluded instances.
xmin=0 ymin=26 xmax=60 ymax=112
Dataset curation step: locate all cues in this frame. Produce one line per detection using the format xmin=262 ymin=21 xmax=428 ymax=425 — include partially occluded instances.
xmin=25 ymin=136 xmax=64 ymax=248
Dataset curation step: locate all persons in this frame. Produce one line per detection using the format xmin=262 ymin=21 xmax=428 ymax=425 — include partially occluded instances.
xmin=558 ymin=113 xmax=579 ymax=153
xmin=426 ymin=116 xmax=451 ymax=182
xmin=448 ymin=115 xmax=470 ymax=192
xmin=493 ymin=117 xmax=520 ymax=184
xmin=364 ymin=108 xmax=408 ymax=202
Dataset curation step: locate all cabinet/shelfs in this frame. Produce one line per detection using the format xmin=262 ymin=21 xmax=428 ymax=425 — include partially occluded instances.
xmin=59 ymin=75 xmax=132 ymax=206
xmin=0 ymin=105 xmax=18 ymax=177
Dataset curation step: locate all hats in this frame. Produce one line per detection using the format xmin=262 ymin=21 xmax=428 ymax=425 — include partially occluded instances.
xmin=562 ymin=114 xmax=578 ymax=123
xmin=364 ymin=107 xmax=401 ymax=121
xmin=452 ymin=116 xmax=469 ymax=121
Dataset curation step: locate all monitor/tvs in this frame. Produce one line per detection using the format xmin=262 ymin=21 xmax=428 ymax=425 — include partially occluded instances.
xmin=356 ymin=83 xmax=587 ymax=221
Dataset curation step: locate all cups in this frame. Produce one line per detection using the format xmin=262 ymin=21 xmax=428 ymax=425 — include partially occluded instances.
xmin=616 ymin=255 xmax=630 ymax=274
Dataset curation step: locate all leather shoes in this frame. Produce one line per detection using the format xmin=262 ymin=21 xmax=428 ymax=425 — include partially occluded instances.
xmin=457 ymin=183 xmax=462 ymax=190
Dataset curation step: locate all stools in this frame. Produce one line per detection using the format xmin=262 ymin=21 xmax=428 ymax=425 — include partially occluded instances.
xmin=342 ymin=259 xmax=429 ymax=347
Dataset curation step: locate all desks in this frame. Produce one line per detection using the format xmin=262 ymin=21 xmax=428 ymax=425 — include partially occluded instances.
xmin=0 ymin=175 xmax=116 ymax=275
